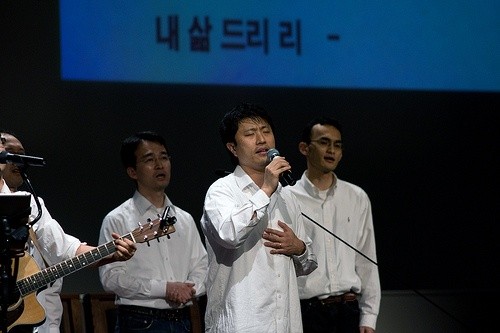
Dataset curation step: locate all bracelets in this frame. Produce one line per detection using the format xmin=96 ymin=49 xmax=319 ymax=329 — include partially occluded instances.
xmin=300 ymin=241 xmax=306 ymax=255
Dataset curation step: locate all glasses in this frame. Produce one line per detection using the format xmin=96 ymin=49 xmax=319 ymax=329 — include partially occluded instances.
xmin=311 ymin=139 xmax=345 ymax=150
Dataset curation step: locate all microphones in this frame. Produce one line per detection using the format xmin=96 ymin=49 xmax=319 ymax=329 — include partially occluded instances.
xmin=267 ymin=148 xmax=296 ymax=186
xmin=0 ymin=150 xmax=46 ymax=167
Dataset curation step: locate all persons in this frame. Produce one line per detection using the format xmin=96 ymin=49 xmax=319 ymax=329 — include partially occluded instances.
xmin=0 ymin=132 xmax=137 ymax=333
xmin=282 ymin=119 xmax=382 ymax=333
xmin=97 ymin=133 xmax=209 ymax=333
xmin=201 ymin=102 xmax=320 ymax=333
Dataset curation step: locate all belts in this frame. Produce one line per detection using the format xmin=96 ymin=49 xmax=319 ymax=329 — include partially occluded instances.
xmin=302 ymin=293 xmax=358 ymax=305
xmin=124 ymin=305 xmax=190 ymax=317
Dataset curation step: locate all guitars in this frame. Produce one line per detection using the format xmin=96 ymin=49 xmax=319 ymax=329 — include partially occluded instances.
xmin=0 ymin=212 xmax=176 ymax=333
